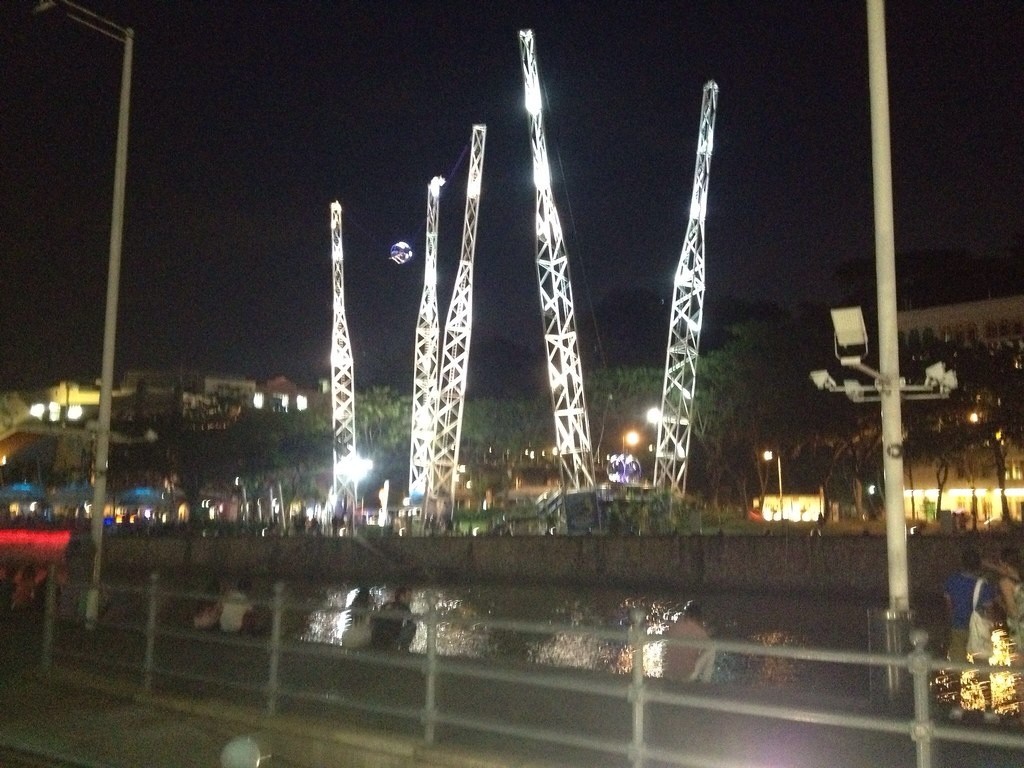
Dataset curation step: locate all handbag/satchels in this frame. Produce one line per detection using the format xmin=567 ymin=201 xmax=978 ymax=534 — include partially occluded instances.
xmin=968 ymin=577 xmax=999 ymax=659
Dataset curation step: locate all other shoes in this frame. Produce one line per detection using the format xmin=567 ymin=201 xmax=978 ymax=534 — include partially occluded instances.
xmin=949 ymin=708 xmax=962 ymax=721
xmin=983 ymin=711 xmax=1000 ymax=725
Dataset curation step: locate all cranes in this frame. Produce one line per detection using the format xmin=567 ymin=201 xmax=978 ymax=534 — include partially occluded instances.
xmin=327 ymin=28 xmax=719 ymax=531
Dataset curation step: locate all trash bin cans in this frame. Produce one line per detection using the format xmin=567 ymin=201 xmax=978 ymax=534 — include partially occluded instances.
xmin=867 ymin=609 xmax=917 ymax=707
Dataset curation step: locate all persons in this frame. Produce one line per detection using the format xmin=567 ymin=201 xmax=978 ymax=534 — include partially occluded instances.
xmin=0 ymin=564 xmax=61 ymax=614
xmin=340 ymin=588 xmax=378 ymax=649
xmin=981 ymin=547 xmax=1024 ymax=650
xmin=193 ymin=582 xmax=222 ymax=630
xmin=312 ymin=518 xmax=318 ymax=526
xmin=943 ymin=544 xmax=1001 ymax=724
xmin=371 ymin=587 xmax=417 ymax=652
xmin=958 ymin=512 xmax=967 ymax=528
xmin=219 ymin=578 xmax=257 ymax=634
xmin=445 ymin=517 xmax=453 ymax=530
xmin=818 ymin=513 xmax=825 ymax=528
xmin=665 ymin=605 xmax=715 ymax=685
xmin=952 ymin=512 xmax=958 ymax=531
xmin=332 ymin=515 xmax=338 ymax=536
